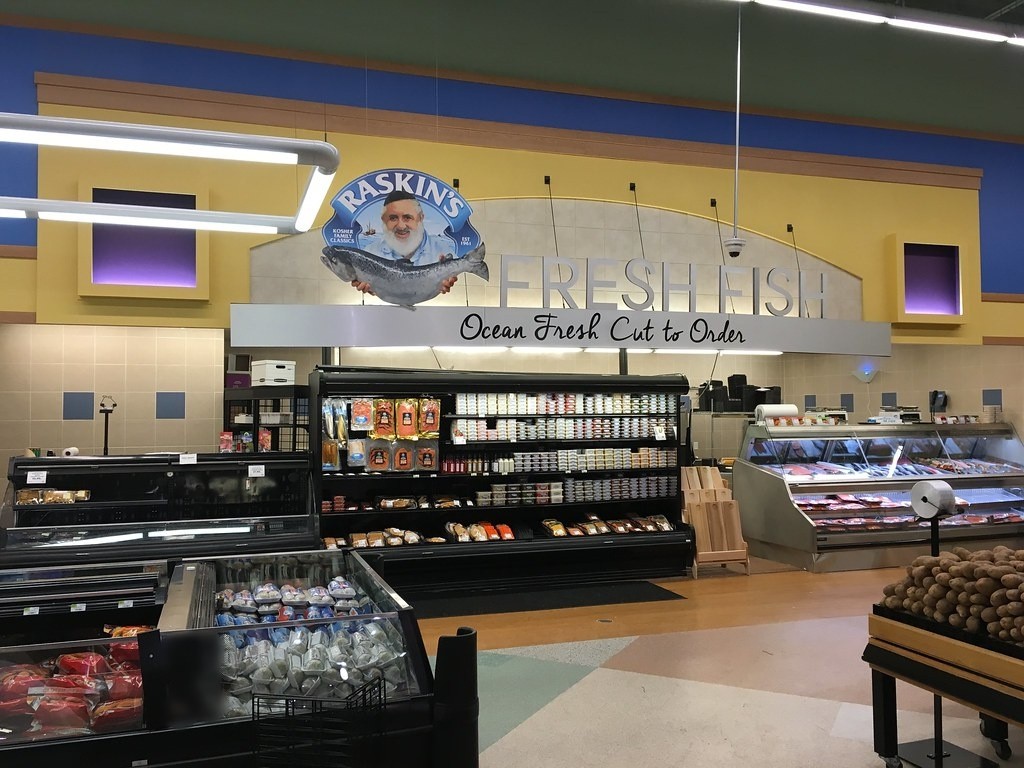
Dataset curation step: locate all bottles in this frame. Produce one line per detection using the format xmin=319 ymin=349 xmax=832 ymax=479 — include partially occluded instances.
xmin=47 ymin=450 xmax=56 ymax=456
xmin=233 ymin=435 xmax=238 ymax=452
xmin=237 ymin=435 xmax=242 ymax=452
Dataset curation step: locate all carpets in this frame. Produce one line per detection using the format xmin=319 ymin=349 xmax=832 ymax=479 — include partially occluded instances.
xmin=403 ymin=581 xmax=689 ymax=620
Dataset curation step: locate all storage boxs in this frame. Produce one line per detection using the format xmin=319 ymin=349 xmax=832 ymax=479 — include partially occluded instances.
xmin=249 ymin=360 xmax=295 ymax=385
xmin=234 ymin=415 xmax=253 ymax=424
xmin=259 ymin=411 xmax=293 ymax=425
xmin=225 ymin=370 xmax=251 ymax=388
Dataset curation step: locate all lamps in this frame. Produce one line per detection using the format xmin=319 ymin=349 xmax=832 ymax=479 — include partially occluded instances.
xmin=0 ymin=112 xmax=338 ymax=236
xmin=754 ymin=0 xmax=1024 ymax=45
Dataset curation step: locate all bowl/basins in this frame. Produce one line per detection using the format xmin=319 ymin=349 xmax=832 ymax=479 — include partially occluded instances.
xmin=980 ymin=405 xmax=1001 ymax=424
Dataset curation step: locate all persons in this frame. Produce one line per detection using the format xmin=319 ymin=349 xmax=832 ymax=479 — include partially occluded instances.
xmin=351 ymin=191 xmax=457 ymax=294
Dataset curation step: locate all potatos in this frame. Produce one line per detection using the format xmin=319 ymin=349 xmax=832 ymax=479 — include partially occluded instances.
xmin=879 ymin=546 xmax=1024 ymax=648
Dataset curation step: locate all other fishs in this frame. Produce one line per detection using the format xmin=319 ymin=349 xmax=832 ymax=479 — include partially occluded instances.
xmin=318 ymin=242 xmax=490 ymax=312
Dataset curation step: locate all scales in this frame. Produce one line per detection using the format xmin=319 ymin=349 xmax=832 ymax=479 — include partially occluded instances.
xmin=879 ymin=405 xmax=922 ymax=424
xmin=804 ymin=407 xmax=849 ymax=425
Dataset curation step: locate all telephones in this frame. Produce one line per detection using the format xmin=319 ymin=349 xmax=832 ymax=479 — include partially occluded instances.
xmin=929 ymin=390 xmax=947 ymax=412
